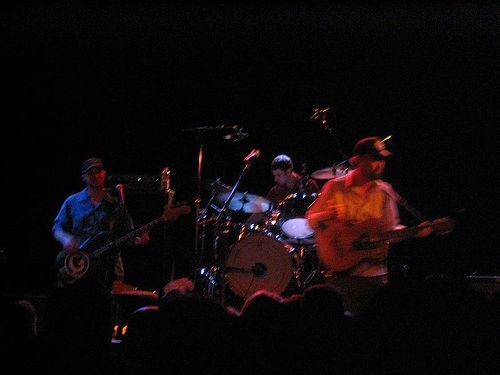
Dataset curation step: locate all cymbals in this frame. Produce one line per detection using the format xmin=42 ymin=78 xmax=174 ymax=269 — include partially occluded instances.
xmin=214 ymin=192 xmax=275 ymax=214
xmin=310 ymin=166 xmax=346 ymax=179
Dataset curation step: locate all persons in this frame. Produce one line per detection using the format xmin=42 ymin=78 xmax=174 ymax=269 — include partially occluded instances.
xmin=50 ymin=158 xmax=149 ymax=295
xmin=0 ymin=259 xmax=500 ymax=375
xmin=303 ymin=136 xmax=433 ymax=318
xmin=243 ymin=154 xmax=320 ymax=287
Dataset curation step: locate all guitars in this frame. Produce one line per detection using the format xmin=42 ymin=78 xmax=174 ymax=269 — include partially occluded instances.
xmin=315 ymin=215 xmax=456 ymax=272
xmin=54 ymin=200 xmax=191 ymax=286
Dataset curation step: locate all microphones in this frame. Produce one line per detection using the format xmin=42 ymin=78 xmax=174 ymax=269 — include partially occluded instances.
xmin=222 ymin=133 xmax=249 ymax=142
xmin=309 ymin=109 xmax=330 ymax=121
xmin=244 ymin=149 xmax=260 ymax=162
xmin=300 ymin=166 xmax=306 ymax=193
xmin=254 ymin=265 xmax=263 ymax=275
xmin=116 ymin=184 xmax=125 ymax=206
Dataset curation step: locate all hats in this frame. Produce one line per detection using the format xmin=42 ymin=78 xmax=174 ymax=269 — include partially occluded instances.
xmin=354 ymin=137 xmax=393 ymax=158
xmin=81 ymin=158 xmax=104 ymax=175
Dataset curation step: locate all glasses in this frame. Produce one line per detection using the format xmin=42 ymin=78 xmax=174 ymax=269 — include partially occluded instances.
xmin=82 ymin=169 xmax=103 ymax=177
xmin=361 ymin=155 xmax=385 ymax=161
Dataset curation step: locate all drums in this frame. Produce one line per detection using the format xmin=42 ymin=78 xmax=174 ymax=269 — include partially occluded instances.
xmin=223 ymin=228 xmax=304 ymax=298
xmin=281 ymin=193 xmax=315 ymax=240
xmin=286 ymin=239 xmax=319 ymax=285
xmin=212 ymin=222 xmax=263 ymax=262
